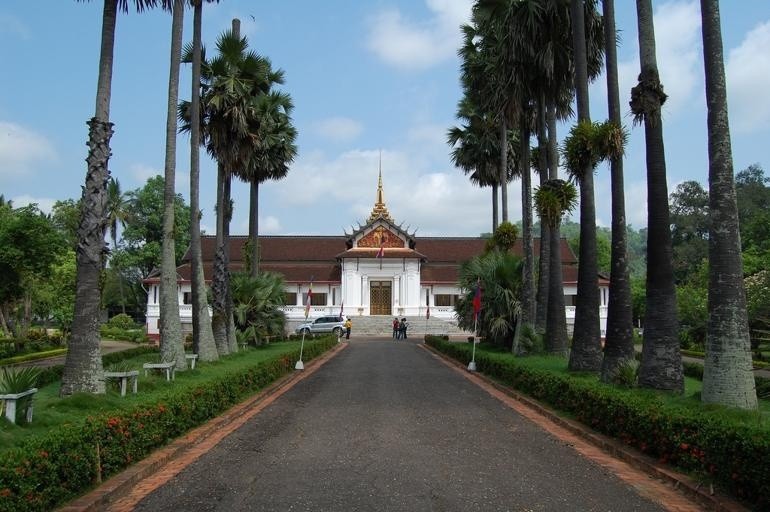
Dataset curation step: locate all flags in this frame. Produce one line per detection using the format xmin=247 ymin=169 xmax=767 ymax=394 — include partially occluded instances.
xmin=472 ymin=287 xmax=481 ymax=321
xmin=305 ymin=284 xmax=312 ymax=318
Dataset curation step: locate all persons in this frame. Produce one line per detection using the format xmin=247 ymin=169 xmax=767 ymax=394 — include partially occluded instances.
xmin=346 ymin=319 xmax=352 ymax=339
xmin=393 ymin=318 xmax=409 ymax=340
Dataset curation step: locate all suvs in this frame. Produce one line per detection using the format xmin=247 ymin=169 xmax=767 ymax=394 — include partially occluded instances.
xmin=295 ymin=315 xmax=351 ymax=338
